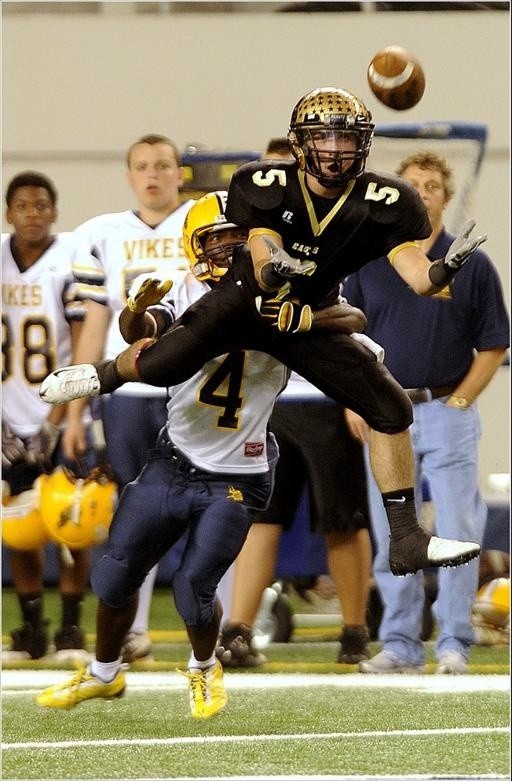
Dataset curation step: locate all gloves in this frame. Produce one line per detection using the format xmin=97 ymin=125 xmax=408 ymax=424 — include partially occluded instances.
xmin=428 ymin=219 xmax=487 ymax=290
xmin=260 ymin=299 xmax=314 ymax=333
xmin=126 ymin=278 xmax=174 ymax=314
xmin=258 ymin=236 xmax=316 ymax=291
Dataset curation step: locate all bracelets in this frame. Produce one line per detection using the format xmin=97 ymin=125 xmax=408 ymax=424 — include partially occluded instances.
xmin=449 ymin=396 xmax=468 ymax=408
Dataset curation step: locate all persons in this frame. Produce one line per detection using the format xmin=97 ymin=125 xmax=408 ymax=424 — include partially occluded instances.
xmin=338 ymin=152 xmax=511 ymax=673
xmin=39 ymin=89 xmax=483 ymax=574
xmin=1 ymin=174 xmax=100 ymax=666
xmin=61 ymin=134 xmax=383 ymax=673
xmin=36 ymin=192 xmax=366 ymax=721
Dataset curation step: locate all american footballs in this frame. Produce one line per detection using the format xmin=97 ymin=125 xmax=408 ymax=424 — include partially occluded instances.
xmin=368 ymin=44 xmax=424 ymax=110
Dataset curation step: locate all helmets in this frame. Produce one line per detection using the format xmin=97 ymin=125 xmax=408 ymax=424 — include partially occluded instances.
xmin=183 ymin=190 xmax=248 ymax=287
xmin=287 ymin=87 xmax=376 ymax=190
xmin=473 ymin=577 xmax=510 ymax=628
xmin=2 ymin=445 xmax=121 ymax=550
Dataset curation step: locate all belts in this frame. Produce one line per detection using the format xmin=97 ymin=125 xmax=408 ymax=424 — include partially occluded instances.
xmin=407 ymin=387 xmax=448 ymax=403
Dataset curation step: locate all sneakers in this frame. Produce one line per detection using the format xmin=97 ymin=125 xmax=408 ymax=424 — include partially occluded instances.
xmin=39 ymin=363 xmax=101 ymax=404
xmin=390 ymin=532 xmax=480 ymax=575
